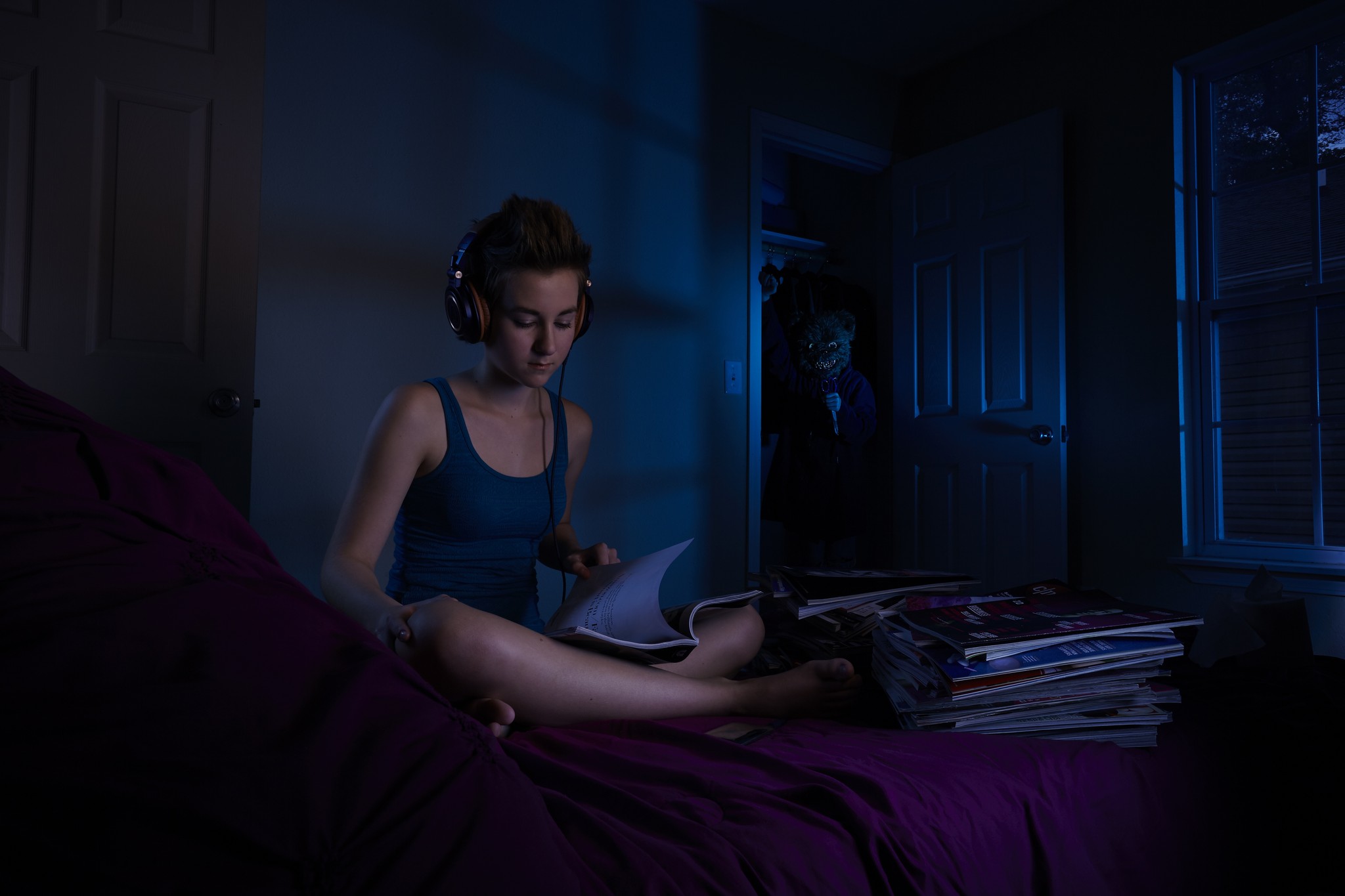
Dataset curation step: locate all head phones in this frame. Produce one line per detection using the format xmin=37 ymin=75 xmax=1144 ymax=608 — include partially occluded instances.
xmin=443 ymin=213 xmax=594 ymax=345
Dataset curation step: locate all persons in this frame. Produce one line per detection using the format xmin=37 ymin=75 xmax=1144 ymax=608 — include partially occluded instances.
xmin=769 ymin=313 xmax=875 ymax=489
xmin=325 ymin=195 xmax=860 ymax=740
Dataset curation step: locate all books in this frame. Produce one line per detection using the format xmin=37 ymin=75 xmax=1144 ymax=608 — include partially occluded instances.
xmin=776 ymin=565 xmax=1202 ymax=747
xmin=548 ymin=543 xmax=762 ymax=662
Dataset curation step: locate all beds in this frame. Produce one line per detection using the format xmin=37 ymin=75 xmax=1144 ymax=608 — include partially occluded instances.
xmin=2 ymin=364 xmax=1345 ymax=896
